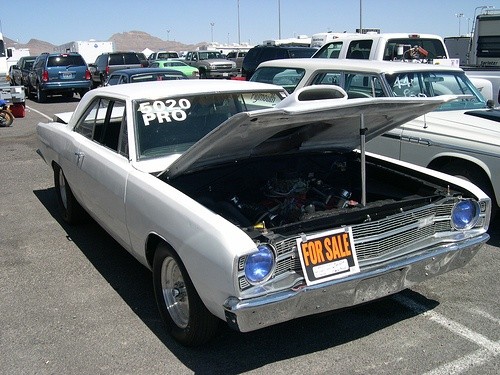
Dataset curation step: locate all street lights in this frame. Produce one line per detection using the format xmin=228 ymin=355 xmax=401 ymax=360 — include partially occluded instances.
xmin=210 ymin=22 xmax=216 ymax=42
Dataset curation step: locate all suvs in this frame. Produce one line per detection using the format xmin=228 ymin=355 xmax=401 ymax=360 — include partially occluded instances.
xmin=248 ymin=56 xmax=499 ymax=232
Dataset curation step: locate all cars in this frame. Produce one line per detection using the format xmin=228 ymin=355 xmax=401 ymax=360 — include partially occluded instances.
xmin=34 ymin=78 xmax=494 ymax=351
xmin=7 ymin=12 xmax=499 ymax=109
